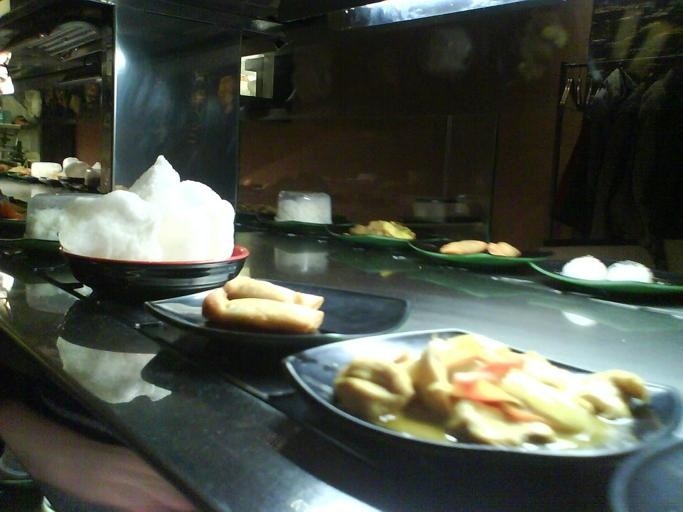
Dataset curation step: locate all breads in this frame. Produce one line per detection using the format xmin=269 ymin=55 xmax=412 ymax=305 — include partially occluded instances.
xmin=440 ymin=240 xmax=487 ymax=254
xmin=487 ymin=242 xmax=521 ymax=256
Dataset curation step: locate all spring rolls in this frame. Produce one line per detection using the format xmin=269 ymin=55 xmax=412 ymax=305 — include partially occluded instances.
xmin=223 ymin=277 xmax=323 ymax=310
xmin=201 ymin=288 xmax=324 ymax=332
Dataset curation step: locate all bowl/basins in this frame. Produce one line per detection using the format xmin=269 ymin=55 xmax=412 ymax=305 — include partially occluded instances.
xmin=56 ymin=243 xmax=251 ymax=303
xmin=50 ymin=289 xmax=170 ymax=353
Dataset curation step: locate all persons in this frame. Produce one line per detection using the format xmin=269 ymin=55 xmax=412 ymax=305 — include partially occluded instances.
xmin=0 ymin=71 xmax=42 ymax=163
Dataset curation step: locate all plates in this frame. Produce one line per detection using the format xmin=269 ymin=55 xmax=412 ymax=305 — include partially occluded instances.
xmin=0 ymin=200 xmax=61 ymax=255
xmin=525 ymin=254 xmax=683 ymax=299
xmin=407 ymin=237 xmax=547 ymax=268
xmin=5 ymin=169 xmax=99 ymax=192
xmin=325 ymin=221 xmax=448 ymax=249
xmin=282 ymin=328 xmax=682 ymax=461
xmin=607 ymin=435 xmax=682 ymax=512
xmin=141 ymin=276 xmax=410 ymax=353
xmin=240 ymin=213 xmax=350 ymax=235
